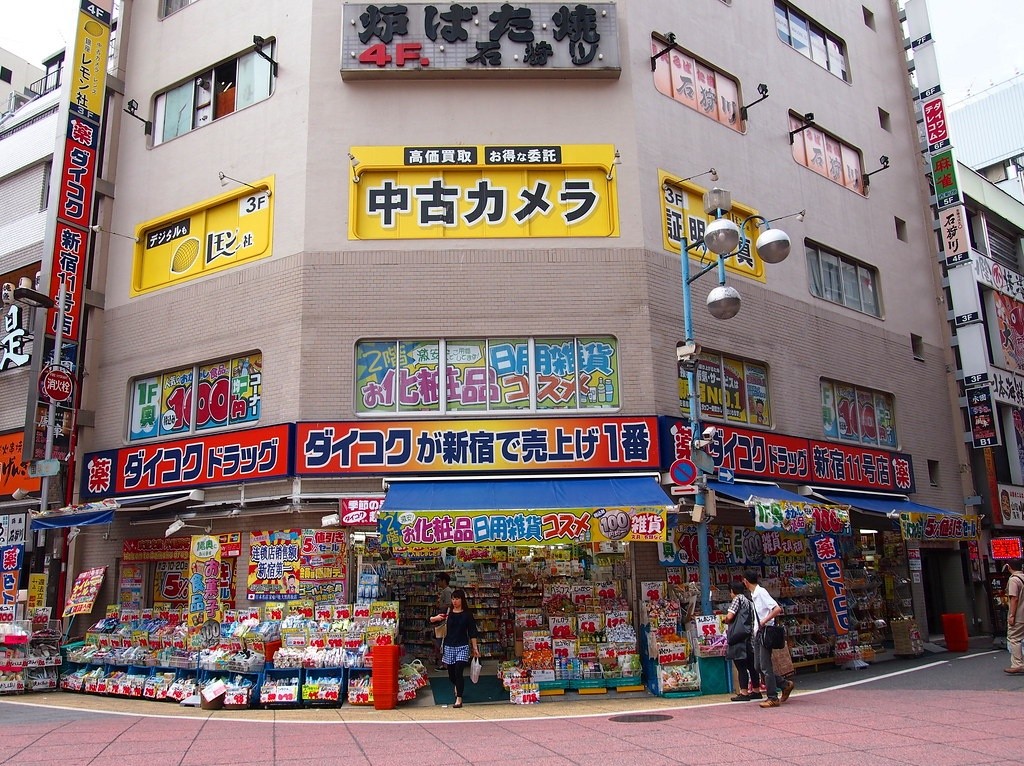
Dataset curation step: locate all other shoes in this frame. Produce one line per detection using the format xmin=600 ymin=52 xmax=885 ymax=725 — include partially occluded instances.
xmin=730 ymin=693 xmax=750 ymax=701
xmin=621 ymin=665 xmax=642 ymax=678
xmin=748 ymin=692 xmax=762 ymax=699
xmin=1003 ymin=667 xmax=1024 ymax=674
xmin=433 ymin=663 xmax=449 ymax=671
xmin=454 ymin=686 xmax=457 ymax=698
xmin=452 ymin=700 xmax=462 ymax=708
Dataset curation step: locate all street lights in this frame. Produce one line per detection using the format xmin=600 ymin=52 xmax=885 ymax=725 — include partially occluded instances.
xmin=12 ymin=280 xmax=67 ymax=577
xmin=671 ymin=186 xmax=793 ymax=618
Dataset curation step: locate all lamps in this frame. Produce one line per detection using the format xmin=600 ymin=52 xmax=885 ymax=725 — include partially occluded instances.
xmin=606 ymin=150 xmax=622 ymax=180
xmin=863 ymin=155 xmax=889 ymax=187
xmin=755 ymin=208 xmax=807 ymax=228
xmin=123 ymin=98 xmax=151 ymax=135
xmin=12 ymin=488 xmax=41 ymax=501
xmin=740 ymin=83 xmax=769 ymax=122
xmin=650 ymin=32 xmax=677 ymax=72
xmin=67 ymin=527 xmax=108 ymax=546
xmin=253 ymin=35 xmax=278 ymax=76
xmin=219 ymin=172 xmax=272 ymax=197
xmin=661 ymin=167 xmax=718 ymax=191
xmin=347 ymin=152 xmax=360 ymax=184
xmin=789 ymin=112 xmax=815 ymax=144
xmin=321 ymin=514 xmax=339 ymax=527
xmin=88 ymin=224 xmax=141 ymax=243
xmin=165 ymin=520 xmax=211 ymax=538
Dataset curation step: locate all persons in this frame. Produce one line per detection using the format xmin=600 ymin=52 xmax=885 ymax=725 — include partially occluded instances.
xmin=428 ymin=590 xmax=480 ymax=708
xmin=742 ymin=569 xmax=794 ymax=708
xmin=721 ymin=581 xmax=763 ymax=701
xmin=1004 ymin=560 xmax=1024 ymax=674
xmin=434 ymin=572 xmax=454 ymax=671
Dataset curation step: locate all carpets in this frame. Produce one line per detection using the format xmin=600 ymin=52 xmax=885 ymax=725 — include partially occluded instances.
xmin=428 ymin=675 xmax=511 ymax=705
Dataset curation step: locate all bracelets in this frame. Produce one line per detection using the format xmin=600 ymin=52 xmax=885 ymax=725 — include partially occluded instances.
xmin=1009 ymin=614 xmax=1015 ymax=618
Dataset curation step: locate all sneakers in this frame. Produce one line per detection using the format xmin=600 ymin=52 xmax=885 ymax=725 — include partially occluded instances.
xmin=780 ymin=681 xmax=795 ymax=702
xmin=759 ymin=699 xmax=780 ymax=708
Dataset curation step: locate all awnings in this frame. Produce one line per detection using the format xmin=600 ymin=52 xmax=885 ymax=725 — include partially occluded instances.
xmin=382 ymin=475 xmax=677 ymax=531
xmin=815 ymin=491 xmax=965 ymax=532
xmin=29 ymin=489 xmax=205 ymax=530
xmin=705 ymin=481 xmax=824 ymax=519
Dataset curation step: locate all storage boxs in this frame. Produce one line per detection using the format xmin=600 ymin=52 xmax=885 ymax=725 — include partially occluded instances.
xmin=891 ymin=620 xmax=924 ymax=655
xmin=372 ymin=645 xmax=401 ymax=711
xmin=942 ymin=612 xmax=968 ymax=653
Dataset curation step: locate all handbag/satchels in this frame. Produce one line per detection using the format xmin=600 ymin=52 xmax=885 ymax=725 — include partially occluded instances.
xmin=434 ymin=607 xmax=450 ymax=638
xmin=469 ymin=655 xmax=482 ymax=684
xmin=760 ymin=625 xmax=786 ymax=650
xmin=726 ymin=597 xmax=753 ymax=646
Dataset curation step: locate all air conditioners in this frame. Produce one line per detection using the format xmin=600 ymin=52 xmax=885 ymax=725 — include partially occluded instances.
xmin=8 ymin=89 xmax=32 ymax=113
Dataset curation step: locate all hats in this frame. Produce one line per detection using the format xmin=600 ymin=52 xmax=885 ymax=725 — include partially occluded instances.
xmin=435 ymin=571 xmax=451 ymax=581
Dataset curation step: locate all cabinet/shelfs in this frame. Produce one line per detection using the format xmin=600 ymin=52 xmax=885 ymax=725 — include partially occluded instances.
xmin=357 ymin=554 xmax=509 ymax=665
xmin=511 ymin=557 xmax=884 ymax=700
xmin=59 ymin=620 xmax=393 ymax=709
xmin=0 ymin=618 xmax=62 ymax=695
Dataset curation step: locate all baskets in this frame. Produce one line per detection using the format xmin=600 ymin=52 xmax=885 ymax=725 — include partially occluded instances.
xmin=556 ymin=668 xmax=582 ymax=680
xmin=584 ymin=672 xmax=602 ymax=679
xmin=642 ymin=600 xmax=730 ymax=696
xmin=60 ymin=619 xmax=400 ymax=706
xmin=603 ymin=672 xmax=622 ymax=679
